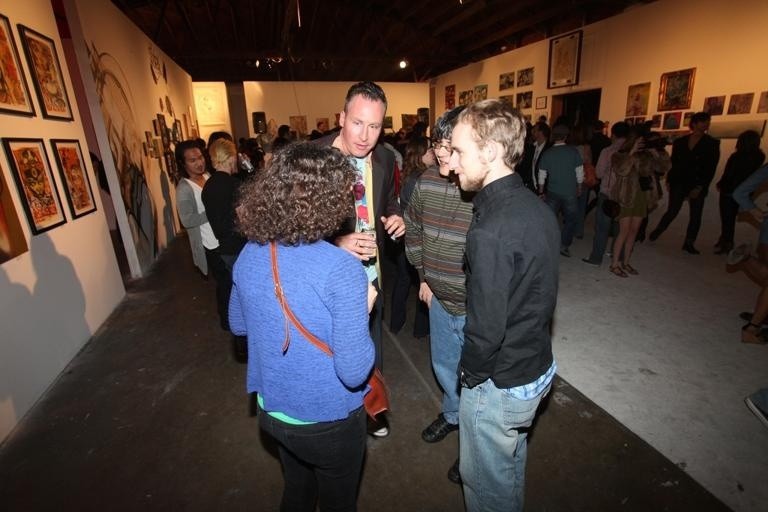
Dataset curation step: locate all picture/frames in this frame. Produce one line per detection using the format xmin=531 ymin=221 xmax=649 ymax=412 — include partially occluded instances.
xmin=656 ymin=66 xmax=698 ymax=113
xmin=0 ymin=13 xmax=36 ymax=116
xmin=15 ymin=24 xmax=73 ymax=122
xmin=3 ymin=137 xmax=68 ymax=234
xmin=50 ymin=138 xmax=97 ymax=219
xmin=547 ymin=29 xmax=584 ymax=89
xmin=144 ymin=106 xmax=198 ymax=181
xmin=534 ymin=97 xmax=547 ymax=107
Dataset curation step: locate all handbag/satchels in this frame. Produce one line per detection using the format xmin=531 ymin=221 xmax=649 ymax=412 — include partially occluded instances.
xmin=602 ymin=199 xmax=621 ymax=219
xmin=364 ymin=363 xmax=391 ymax=421
xmin=583 ymin=144 xmax=600 ymax=187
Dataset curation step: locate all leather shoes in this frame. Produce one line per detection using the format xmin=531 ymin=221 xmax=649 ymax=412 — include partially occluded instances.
xmin=682 ymin=241 xmax=700 ymax=255
xmin=422 ymin=414 xmax=459 ymax=442
xmin=447 ymin=457 xmax=462 ymax=484
xmin=367 ymin=417 xmax=389 ymax=437
xmin=649 ymin=230 xmax=662 ymax=240
xmin=560 ymin=248 xmax=639 ymax=276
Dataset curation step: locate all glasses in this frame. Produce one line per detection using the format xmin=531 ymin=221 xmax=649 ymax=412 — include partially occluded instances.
xmin=431 ymin=142 xmax=452 ymax=152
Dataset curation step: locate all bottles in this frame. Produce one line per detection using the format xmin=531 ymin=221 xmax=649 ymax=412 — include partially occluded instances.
xmin=360 ymin=227 xmax=378 ymax=265
xmin=238 ymin=152 xmax=254 ymax=173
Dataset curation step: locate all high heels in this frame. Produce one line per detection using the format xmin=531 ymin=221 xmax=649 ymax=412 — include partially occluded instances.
xmin=741 ymin=324 xmax=767 ymax=344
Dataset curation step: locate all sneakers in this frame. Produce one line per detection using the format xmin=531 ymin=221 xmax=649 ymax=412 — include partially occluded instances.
xmin=743 ymin=395 xmax=768 ymax=429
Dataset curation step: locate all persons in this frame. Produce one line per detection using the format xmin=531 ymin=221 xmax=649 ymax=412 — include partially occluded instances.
xmin=228 ymin=78 xmax=561 ymax=511
xmin=175 ymin=129 xmax=252 ymax=363
xmin=515 ymin=113 xmax=767 ymax=426
